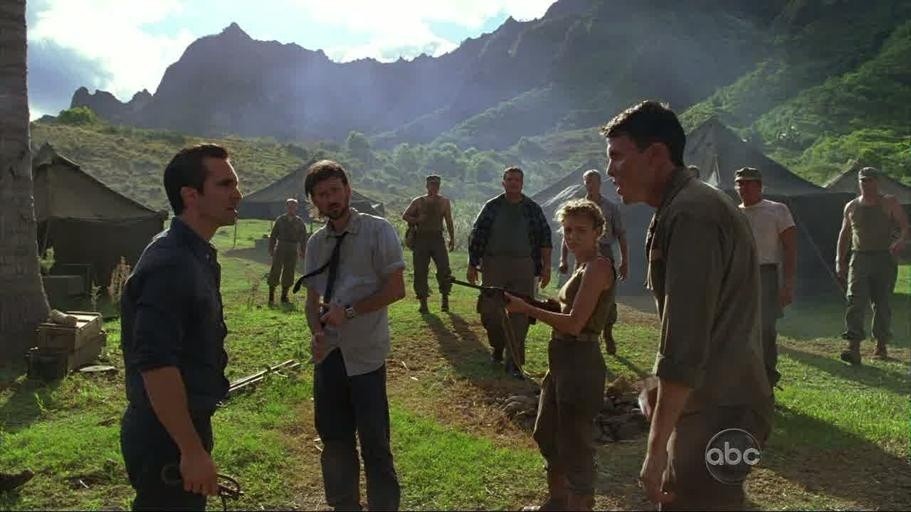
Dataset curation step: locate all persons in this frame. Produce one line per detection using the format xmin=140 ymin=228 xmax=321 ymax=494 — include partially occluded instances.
xmin=557 ymin=169 xmax=629 ymax=354
xmin=598 ymin=98 xmax=776 ymax=512
xmin=119 ymin=142 xmax=243 ymax=512
xmin=401 ymin=174 xmax=455 ymax=315
xmin=266 ymin=197 xmax=306 ymax=306
xmin=503 ymin=197 xmax=617 ymax=512
xmin=835 ymin=166 xmax=911 ymax=364
xmin=733 ymin=167 xmax=799 ymax=388
xmin=688 ymin=164 xmax=702 ymax=179
xmin=292 ymin=158 xmax=407 ymax=511
xmin=466 ymin=167 xmax=552 ymax=381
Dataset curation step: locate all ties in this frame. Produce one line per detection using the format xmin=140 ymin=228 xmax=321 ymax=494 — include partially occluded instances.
xmin=292 ymin=230 xmax=347 ymax=329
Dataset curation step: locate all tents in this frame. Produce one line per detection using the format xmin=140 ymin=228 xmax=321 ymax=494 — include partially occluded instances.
xmin=527 ymin=111 xmax=911 ymax=291
xmin=32 ymin=137 xmax=170 ymax=311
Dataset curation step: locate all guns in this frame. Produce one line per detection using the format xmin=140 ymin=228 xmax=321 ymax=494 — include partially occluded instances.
xmin=445 ymin=277 xmax=560 ymax=314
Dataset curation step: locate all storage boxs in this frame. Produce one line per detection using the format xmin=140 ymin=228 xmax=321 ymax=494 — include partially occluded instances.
xmin=31 ymin=308 xmax=106 ymax=356
xmin=21 ymin=329 xmax=108 ymax=375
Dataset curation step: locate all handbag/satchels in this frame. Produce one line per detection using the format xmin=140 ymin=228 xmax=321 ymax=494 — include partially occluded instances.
xmin=405 ymin=220 xmax=420 ymax=253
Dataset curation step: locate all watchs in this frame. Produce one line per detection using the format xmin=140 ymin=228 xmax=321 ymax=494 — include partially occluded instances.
xmin=345 ymin=306 xmax=356 ymax=320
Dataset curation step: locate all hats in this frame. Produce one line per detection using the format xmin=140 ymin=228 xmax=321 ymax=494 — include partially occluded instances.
xmin=858 ymin=166 xmax=879 ymax=181
xmin=735 ymin=167 xmax=761 ymax=183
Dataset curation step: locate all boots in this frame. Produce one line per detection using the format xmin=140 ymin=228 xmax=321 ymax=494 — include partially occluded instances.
xmin=872 ymin=340 xmax=888 ymax=361
xmin=841 ymin=340 xmax=861 ymax=364
